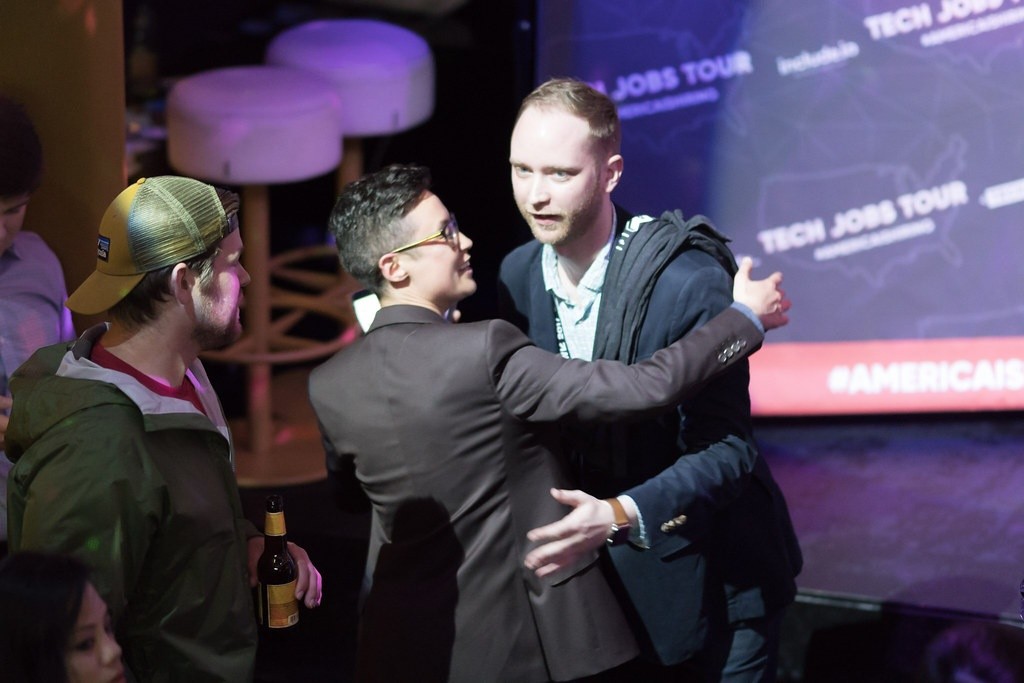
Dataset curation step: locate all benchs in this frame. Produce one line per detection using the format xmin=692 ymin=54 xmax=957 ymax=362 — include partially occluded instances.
xmin=768 ymin=589 xmax=1024 ymax=683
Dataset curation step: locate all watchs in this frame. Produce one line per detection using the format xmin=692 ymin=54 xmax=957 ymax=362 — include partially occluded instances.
xmin=606 ymin=498 xmax=631 ymax=547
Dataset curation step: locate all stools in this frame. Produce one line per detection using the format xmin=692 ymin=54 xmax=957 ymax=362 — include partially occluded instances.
xmin=169 ymin=67 xmax=360 ymax=486
xmin=270 ymin=19 xmax=434 ymax=308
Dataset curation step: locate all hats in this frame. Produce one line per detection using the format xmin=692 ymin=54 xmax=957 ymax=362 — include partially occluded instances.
xmin=66 ymin=174 xmax=239 ymax=316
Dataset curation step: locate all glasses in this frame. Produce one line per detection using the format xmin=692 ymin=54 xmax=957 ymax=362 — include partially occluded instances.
xmin=390 ymin=214 xmax=457 ymax=257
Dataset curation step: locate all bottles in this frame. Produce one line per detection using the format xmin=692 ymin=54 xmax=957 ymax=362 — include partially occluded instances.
xmin=255 ymin=493 xmax=301 ymax=646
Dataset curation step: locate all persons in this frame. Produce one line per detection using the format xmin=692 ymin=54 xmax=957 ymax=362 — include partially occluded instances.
xmin=308 ymin=78 xmax=804 ymax=683
xmin=0 ymin=102 xmax=323 ymax=683
